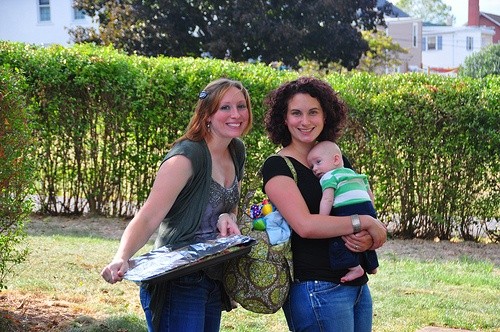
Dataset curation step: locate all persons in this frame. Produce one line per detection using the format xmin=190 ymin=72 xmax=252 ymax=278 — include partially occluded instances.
xmin=101 ymin=78 xmax=253 ymax=331
xmin=305 ymin=141 xmax=380 ymax=285
xmin=261 ymin=77 xmax=388 ymax=332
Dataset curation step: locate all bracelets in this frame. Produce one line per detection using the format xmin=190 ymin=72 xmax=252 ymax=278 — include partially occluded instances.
xmin=217 ymin=212 xmax=231 ymax=223
xmin=350 ymin=213 xmax=362 ymax=234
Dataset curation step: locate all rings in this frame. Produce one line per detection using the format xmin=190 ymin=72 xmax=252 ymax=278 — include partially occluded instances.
xmin=355 ymin=243 xmax=360 ymax=250
xmin=106 ymin=278 xmax=109 ymax=282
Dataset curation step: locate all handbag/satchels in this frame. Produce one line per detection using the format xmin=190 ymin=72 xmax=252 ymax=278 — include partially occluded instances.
xmin=220 ymin=153 xmax=299 ymax=315
